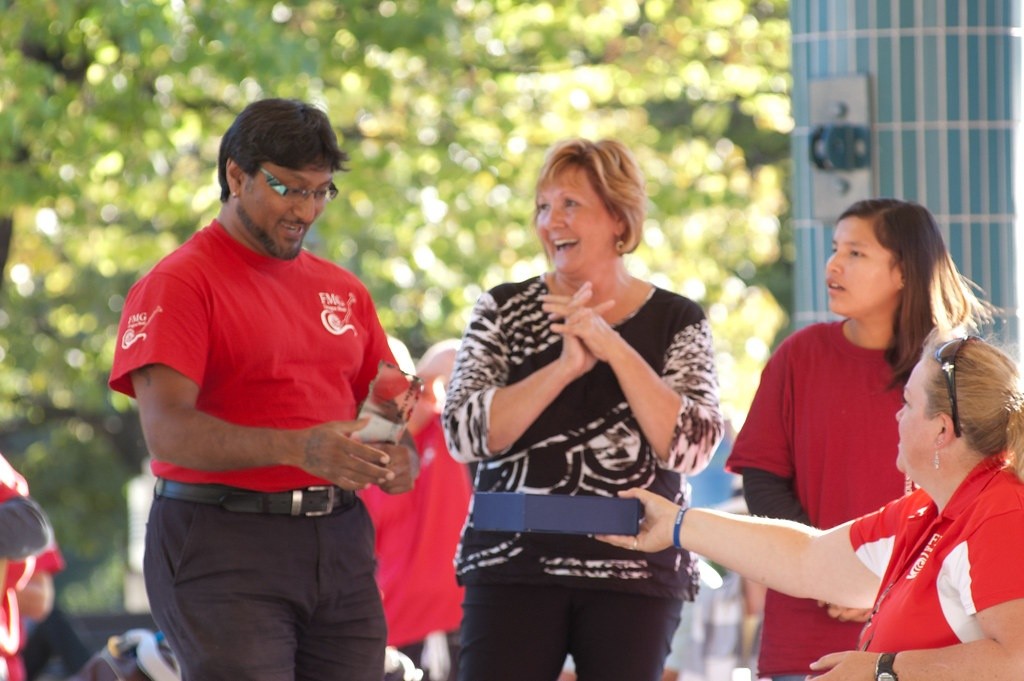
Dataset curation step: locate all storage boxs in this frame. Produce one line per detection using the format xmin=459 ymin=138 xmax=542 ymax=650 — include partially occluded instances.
xmin=472 ymin=490 xmax=640 ymax=537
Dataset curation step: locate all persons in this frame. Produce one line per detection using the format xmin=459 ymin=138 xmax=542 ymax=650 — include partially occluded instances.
xmin=0 ymin=98 xmax=474 ymax=681
xmin=595 ymin=198 xmax=1023 ymax=681
xmin=440 ymin=136 xmax=724 ymax=681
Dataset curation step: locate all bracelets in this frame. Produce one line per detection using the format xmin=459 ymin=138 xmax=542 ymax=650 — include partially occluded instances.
xmin=875 ymin=652 xmax=883 ymax=681
xmin=673 ymin=506 xmax=690 ymax=548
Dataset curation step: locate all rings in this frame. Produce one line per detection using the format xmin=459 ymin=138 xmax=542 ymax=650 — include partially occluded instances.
xmin=633 ymin=536 xmax=638 ymax=550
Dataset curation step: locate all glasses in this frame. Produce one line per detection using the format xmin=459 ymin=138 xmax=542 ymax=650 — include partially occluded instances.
xmin=258 ymin=167 xmax=339 ymax=203
xmin=935 ymin=335 xmax=985 ymax=438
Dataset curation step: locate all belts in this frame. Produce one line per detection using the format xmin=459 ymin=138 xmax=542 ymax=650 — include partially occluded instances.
xmin=156 ymin=478 xmax=354 ymax=516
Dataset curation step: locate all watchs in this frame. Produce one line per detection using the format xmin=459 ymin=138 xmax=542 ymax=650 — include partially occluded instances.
xmin=876 ymin=651 xmax=900 ymax=681
